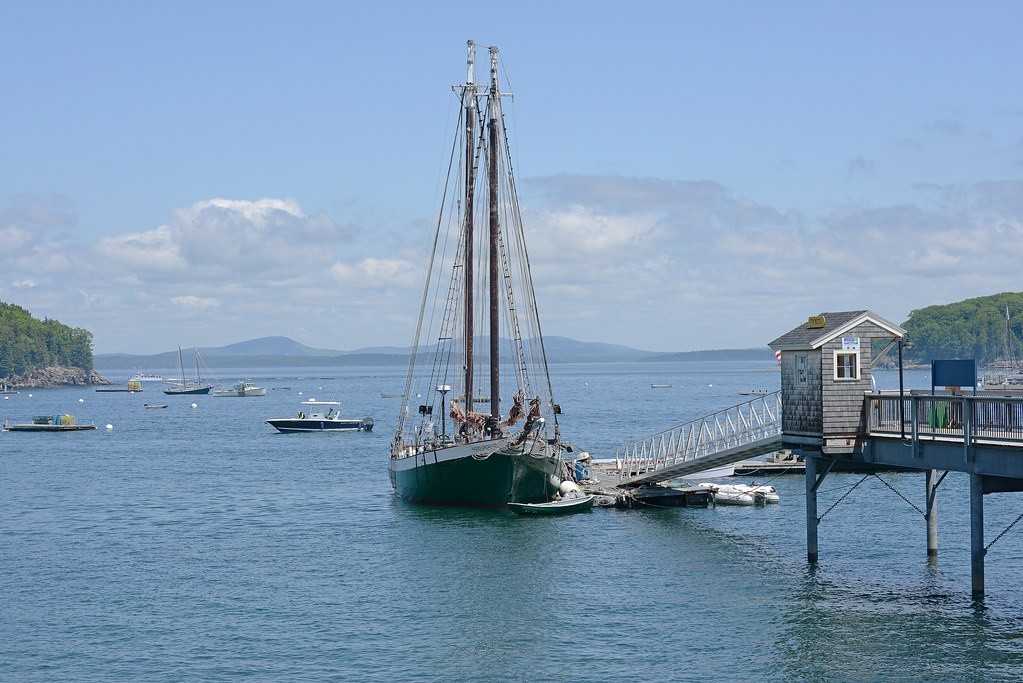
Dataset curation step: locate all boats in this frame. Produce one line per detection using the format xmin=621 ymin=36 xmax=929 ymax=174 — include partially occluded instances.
xmin=96 ymin=383 xmax=143 ymax=393
xmin=3 ymin=414 xmax=97 ymax=431
xmin=507 ymin=494 xmax=595 ymax=515
xmin=128 ymin=371 xmax=162 ymax=382
xmin=451 ymin=395 xmax=502 ymax=402
xmin=213 ymin=375 xmax=266 ymax=397
xmin=976 ymin=307 xmax=1023 ymax=390
xmin=0 ymin=384 xmax=19 ymax=394
xmin=574 ymin=448 xmax=806 ymax=508
xmin=266 ymin=381 xmax=374 ymax=432
xmin=143 ymin=403 xmax=169 ymax=409
xmin=651 ymin=384 xmax=671 ymax=389
xmin=739 ymin=390 xmax=769 ymax=395
xmin=381 ymin=392 xmax=405 ymax=398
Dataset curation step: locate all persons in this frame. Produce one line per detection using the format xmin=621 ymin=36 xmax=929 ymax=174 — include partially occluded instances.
xmin=299 ymin=411 xmax=305 ymax=419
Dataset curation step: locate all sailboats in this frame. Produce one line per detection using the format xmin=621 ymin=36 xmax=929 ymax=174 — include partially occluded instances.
xmin=163 ymin=342 xmax=219 ymax=395
xmin=389 ymin=40 xmax=573 ymax=509
